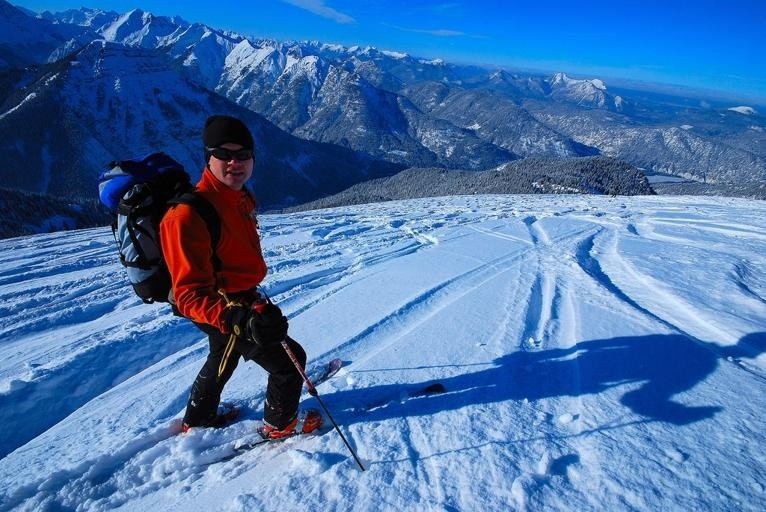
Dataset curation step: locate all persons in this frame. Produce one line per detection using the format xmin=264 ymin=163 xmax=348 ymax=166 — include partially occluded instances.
xmin=158 ymin=115 xmax=322 ymax=437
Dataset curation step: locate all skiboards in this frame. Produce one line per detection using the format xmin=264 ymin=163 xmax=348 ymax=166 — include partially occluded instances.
xmin=162 ymin=358 xmax=446 ymax=475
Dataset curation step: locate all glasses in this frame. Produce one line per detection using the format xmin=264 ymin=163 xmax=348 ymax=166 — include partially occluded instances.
xmin=204 ymin=146 xmax=254 ymax=161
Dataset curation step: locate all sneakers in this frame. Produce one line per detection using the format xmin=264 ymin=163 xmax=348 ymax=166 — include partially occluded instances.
xmin=263 ymin=409 xmax=321 ymax=438
xmin=182 ymin=403 xmax=240 ymax=432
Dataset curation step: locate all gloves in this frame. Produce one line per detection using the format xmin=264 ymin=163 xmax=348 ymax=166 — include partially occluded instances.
xmin=230 ymin=309 xmax=288 ymax=347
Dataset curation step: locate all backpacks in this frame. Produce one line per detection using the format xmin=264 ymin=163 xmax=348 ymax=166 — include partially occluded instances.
xmin=96 ymin=151 xmax=221 ymax=303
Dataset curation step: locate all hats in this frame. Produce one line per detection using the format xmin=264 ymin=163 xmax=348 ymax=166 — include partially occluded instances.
xmin=204 ymin=115 xmax=252 ymax=149
